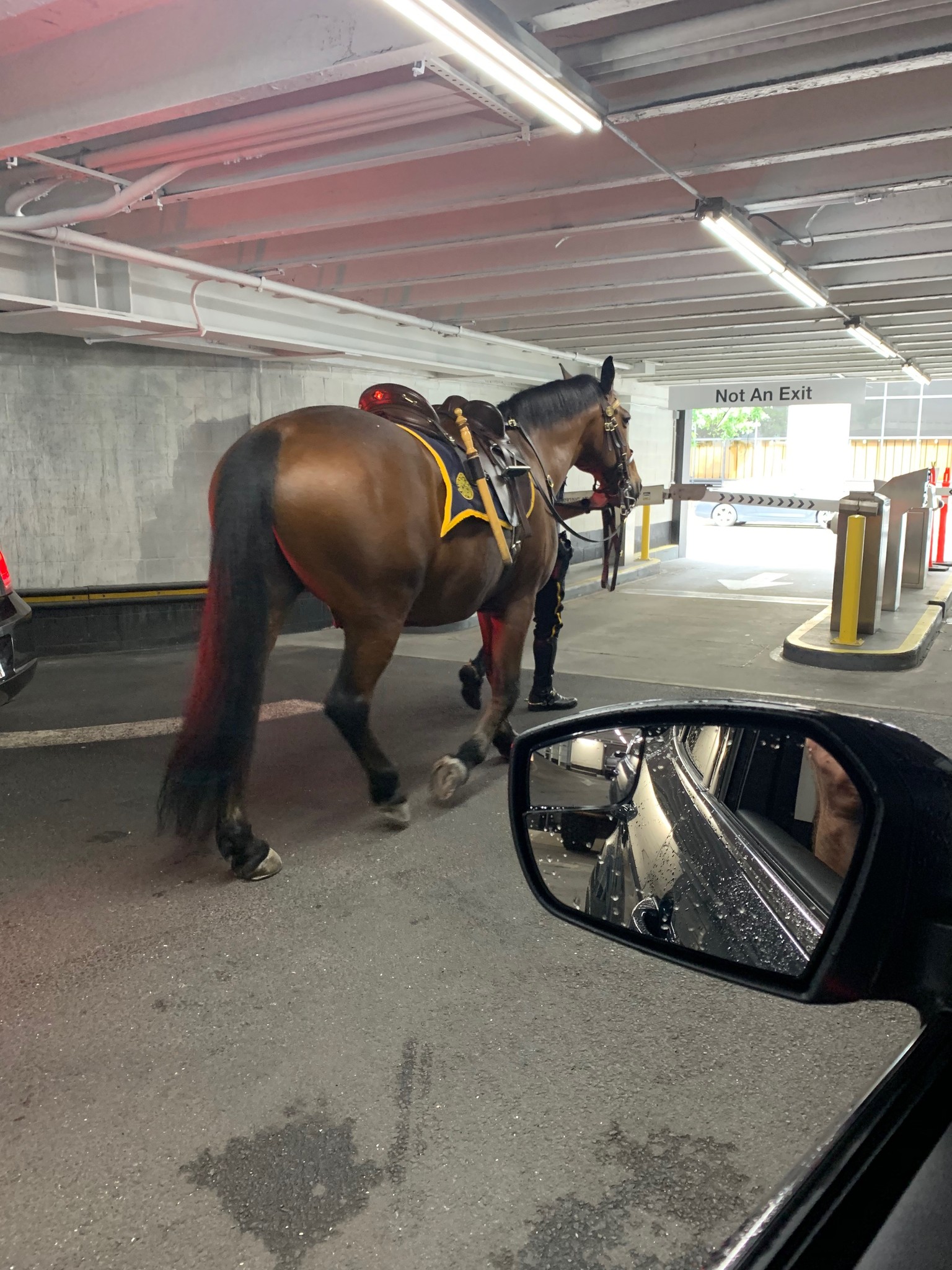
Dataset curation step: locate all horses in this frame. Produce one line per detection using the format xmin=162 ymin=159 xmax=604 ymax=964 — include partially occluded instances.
xmin=156 ymin=356 xmax=643 ymax=881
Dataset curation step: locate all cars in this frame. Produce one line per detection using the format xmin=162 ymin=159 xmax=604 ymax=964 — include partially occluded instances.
xmin=604 ymin=750 xmax=626 ymax=780
xmin=608 ymin=734 xmax=645 ymax=805
xmin=0 ymin=550 xmax=40 ymax=708
xmin=695 ymin=500 xmax=839 ymax=529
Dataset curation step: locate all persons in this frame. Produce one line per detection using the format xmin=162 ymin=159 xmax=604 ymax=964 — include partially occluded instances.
xmin=459 ymin=478 xmax=578 ymax=713
xmin=805 ymin=738 xmax=864 ymax=879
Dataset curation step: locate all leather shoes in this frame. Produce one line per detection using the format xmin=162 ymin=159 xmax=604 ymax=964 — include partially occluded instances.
xmin=457 ymin=659 xmax=483 ymax=712
xmin=525 ymin=686 xmax=578 ymax=711
